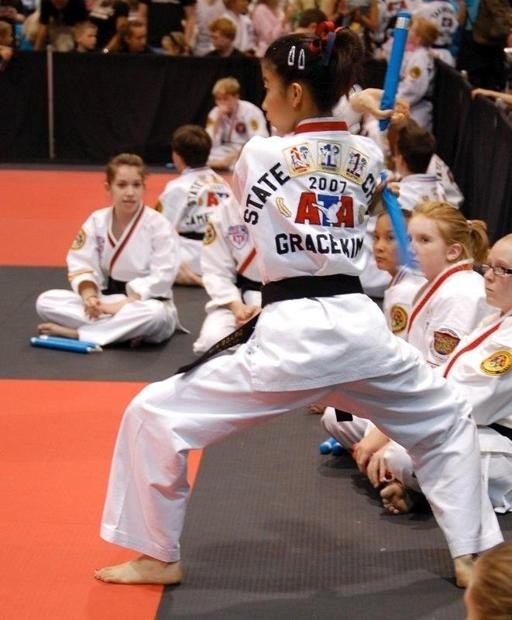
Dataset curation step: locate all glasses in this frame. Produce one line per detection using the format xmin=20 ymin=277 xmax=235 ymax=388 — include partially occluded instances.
xmin=481 ymin=263 xmax=512 ymax=276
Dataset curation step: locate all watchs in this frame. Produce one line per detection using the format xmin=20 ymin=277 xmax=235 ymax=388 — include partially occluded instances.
xmin=100 ymin=47 xmax=112 ymax=56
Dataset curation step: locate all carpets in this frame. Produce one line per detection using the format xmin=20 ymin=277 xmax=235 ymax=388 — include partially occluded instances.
xmin=1 ymin=264 xmax=210 ymax=382
xmin=153 ymin=405 xmax=512 ymax=620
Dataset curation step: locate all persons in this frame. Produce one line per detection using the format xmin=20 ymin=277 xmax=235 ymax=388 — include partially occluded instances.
xmin=319 ymin=200 xmax=501 ymax=474
xmin=204 ymin=19 xmax=245 ymax=57
xmin=471 ymin=0 xmax=512 ymax=89
xmin=111 ymin=16 xmax=161 ymax=55
xmin=73 ymin=20 xmax=98 ymax=54
xmin=384 ymin=117 xmax=464 ymax=213
xmin=292 ymin=9 xmax=327 ymax=34
xmin=221 ymin=0 xmax=255 ymax=57
xmin=33 ymin=0 xmax=87 ymax=51
xmin=88 ymin=0 xmax=131 ymax=57
xmin=165 ymin=77 xmax=270 ymax=172
xmin=290 ymin=0 xmax=335 ymax=30
xmin=94 ymin=26 xmax=511 ymax=587
xmin=462 ymin=545 xmax=512 ymax=615
xmin=192 ymin=196 xmax=265 ymax=356
xmin=415 ymin=0 xmax=457 ymax=69
xmin=308 ymin=207 xmax=430 ymax=415
xmin=252 ymin=0 xmax=290 ymax=57
xmin=153 ymin=124 xmax=243 ymax=288
xmin=142 ymin=1 xmax=193 ymax=57
xmin=0 ymin=0 xmax=28 ymax=48
xmin=319 ymin=0 xmax=358 ymax=27
xmin=21 ymin=0 xmax=42 ymax=43
xmin=0 ymin=22 xmax=14 ymax=71
xmin=193 ymin=0 xmax=229 ymax=57
xmin=367 ymin=232 xmax=512 ymax=515
xmin=470 ymin=28 xmax=512 ymax=118
xmin=35 ymin=153 xmax=181 ymax=348
xmin=358 ymin=122 xmax=448 ymax=298
xmin=448 ymin=0 xmax=480 ymax=70
xmin=356 ymin=17 xmax=440 ymax=131
xmin=366 ymin=0 xmax=410 ymax=46
xmin=336 ymin=0 xmax=379 ymax=32
